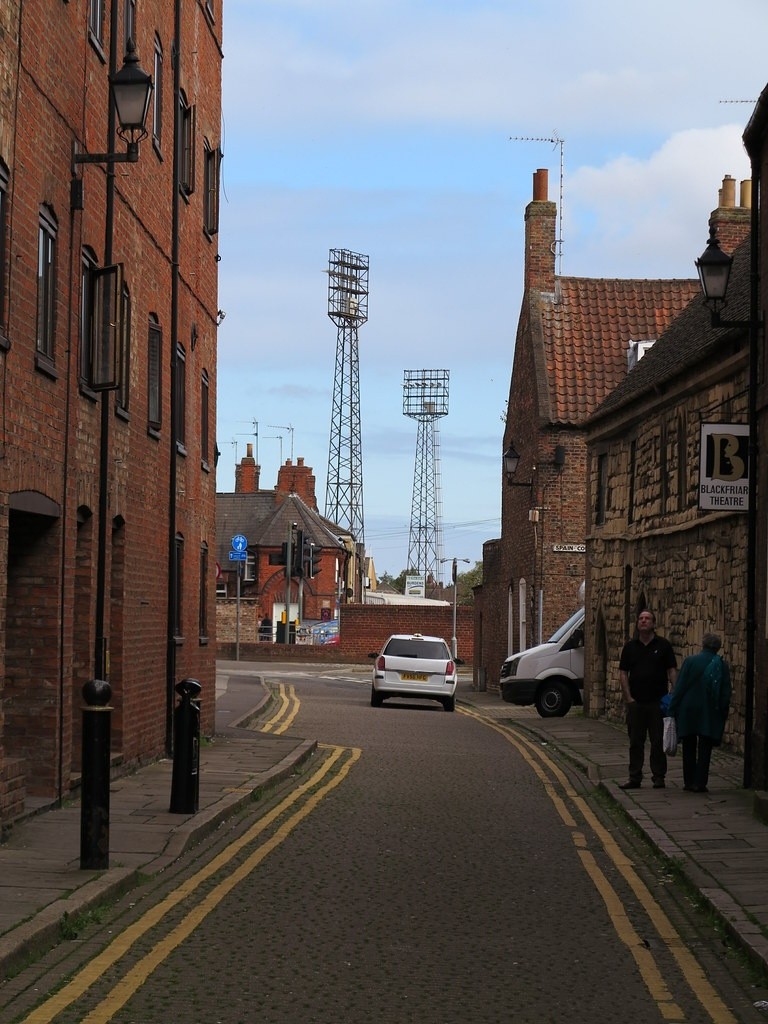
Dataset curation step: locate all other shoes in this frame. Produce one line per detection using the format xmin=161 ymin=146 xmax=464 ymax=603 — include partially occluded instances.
xmin=616 ymin=780 xmax=642 ymax=789
xmin=694 ymin=786 xmax=709 ymax=793
xmin=682 ymin=784 xmax=694 ymax=791
xmin=652 ymin=779 xmax=666 ymax=788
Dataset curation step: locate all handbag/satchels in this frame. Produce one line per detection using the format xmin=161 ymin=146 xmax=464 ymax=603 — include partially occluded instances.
xmin=662 ymin=717 xmax=678 ymax=758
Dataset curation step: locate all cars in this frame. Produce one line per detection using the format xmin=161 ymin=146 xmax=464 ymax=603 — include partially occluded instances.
xmin=368 ymin=630 xmax=464 ymax=712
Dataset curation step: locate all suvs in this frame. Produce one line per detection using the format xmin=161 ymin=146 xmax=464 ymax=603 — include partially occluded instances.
xmin=499 ymin=606 xmax=587 ymax=717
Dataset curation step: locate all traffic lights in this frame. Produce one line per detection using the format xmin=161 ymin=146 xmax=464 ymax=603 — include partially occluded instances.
xmin=294 ymin=528 xmax=313 ymax=574
xmin=310 ymin=542 xmax=323 ymax=580
xmin=282 ymin=541 xmax=299 ymax=577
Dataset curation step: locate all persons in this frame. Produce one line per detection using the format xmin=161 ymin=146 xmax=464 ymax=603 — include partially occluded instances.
xmin=617 ymin=608 xmax=678 ymax=789
xmin=667 ymin=633 xmax=733 ymax=794
